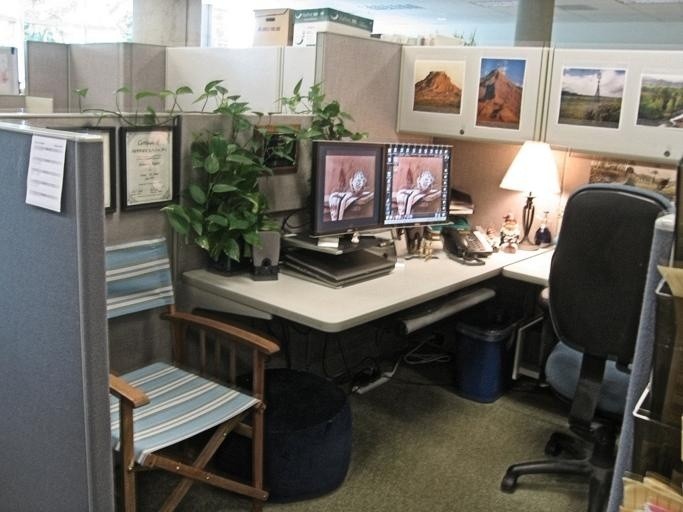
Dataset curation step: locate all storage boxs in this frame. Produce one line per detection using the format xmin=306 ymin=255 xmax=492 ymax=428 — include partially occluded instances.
xmin=648 ymin=231 xmax=682 ymax=433
xmin=290 ymin=7 xmax=375 ymax=48
xmin=250 ymin=8 xmax=293 ymax=46
xmin=631 ymin=380 xmax=682 ymax=483
xmin=449 ymin=299 xmax=531 ymax=405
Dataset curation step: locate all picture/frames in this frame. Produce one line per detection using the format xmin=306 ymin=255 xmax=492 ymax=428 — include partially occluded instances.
xmin=250 ymin=122 xmax=303 ymax=178
xmin=45 ymin=125 xmax=117 ymax=215
xmin=118 ymin=124 xmax=182 ymax=214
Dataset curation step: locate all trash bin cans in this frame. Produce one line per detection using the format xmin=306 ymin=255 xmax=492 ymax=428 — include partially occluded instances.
xmin=452 ymin=309 xmax=528 ymax=403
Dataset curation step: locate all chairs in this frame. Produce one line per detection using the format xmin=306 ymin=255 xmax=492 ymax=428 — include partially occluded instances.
xmin=500 ymin=182 xmax=676 ymax=512
xmin=103 ymin=235 xmax=285 ymax=511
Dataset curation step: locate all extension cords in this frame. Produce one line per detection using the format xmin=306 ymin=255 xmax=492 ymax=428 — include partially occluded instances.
xmin=351 ymin=371 xmax=393 ymax=395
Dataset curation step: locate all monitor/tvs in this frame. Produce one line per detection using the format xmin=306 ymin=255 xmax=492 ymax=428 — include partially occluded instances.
xmin=385 ymin=142 xmax=454 ymax=260
xmin=309 ymin=140 xmax=384 ymax=245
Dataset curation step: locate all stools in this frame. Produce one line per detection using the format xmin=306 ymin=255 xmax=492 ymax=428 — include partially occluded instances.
xmin=216 ymin=366 xmax=355 ymax=507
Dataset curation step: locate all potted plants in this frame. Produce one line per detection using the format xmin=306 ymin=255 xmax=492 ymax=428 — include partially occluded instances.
xmin=70 ymin=75 xmax=372 ymax=277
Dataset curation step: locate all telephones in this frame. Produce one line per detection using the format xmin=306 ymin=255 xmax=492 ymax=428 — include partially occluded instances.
xmin=440 ymin=223 xmax=494 ymax=267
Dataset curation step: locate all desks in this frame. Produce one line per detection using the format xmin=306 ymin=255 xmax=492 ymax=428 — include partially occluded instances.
xmin=178 ymin=225 xmax=557 ymax=387
xmin=512 ymin=241 xmax=554 ymax=392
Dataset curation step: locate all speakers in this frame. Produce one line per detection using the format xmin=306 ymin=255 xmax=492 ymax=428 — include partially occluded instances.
xmin=251 ymin=230 xmax=280 ymax=282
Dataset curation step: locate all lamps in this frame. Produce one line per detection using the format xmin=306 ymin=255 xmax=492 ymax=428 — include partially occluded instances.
xmin=498 ymin=138 xmax=563 ymax=253
